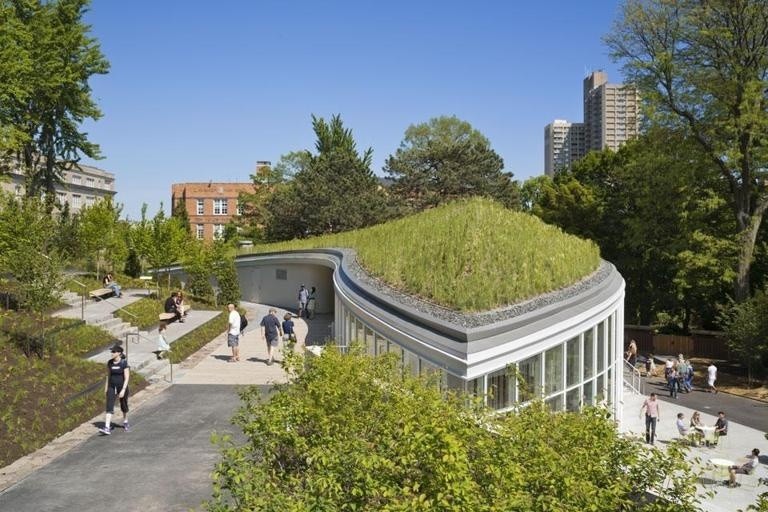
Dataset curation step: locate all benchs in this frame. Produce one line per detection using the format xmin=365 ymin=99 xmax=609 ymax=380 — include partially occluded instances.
xmin=157 ymin=304 xmax=190 ymax=325
xmin=89 ymin=284 xmax=121 ymax=302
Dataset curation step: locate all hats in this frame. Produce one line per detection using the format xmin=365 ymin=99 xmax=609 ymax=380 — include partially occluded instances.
xmin=109 ymin=346 xmax=123 ymax=352
xmin=301 ymin=284 xmax=305 ymax=287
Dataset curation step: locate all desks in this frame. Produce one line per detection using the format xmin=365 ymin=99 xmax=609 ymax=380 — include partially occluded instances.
xmin=709 ymin=458 xmax=735 ymax=477
xmin=695 ymin=426 xmax=716 ymax=446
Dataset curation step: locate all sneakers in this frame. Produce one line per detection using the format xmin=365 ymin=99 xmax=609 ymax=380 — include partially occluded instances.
xmin=123 ymin=423 xmax=130 ymax=432
xmin=99 ymin=427 xmax=111 ymax=435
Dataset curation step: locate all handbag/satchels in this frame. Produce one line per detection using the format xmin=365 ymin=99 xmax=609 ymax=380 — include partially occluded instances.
xmin=240 ymin=315 xmax=248 ymax=330
xmin=289 ymin=335 xmax=297 ymax=342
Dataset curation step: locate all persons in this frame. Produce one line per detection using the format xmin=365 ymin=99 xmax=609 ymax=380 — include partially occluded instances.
xmin=260 ymin=309 xmax=282 ymax=365
xmin=298 ymin=285 xmax=308 ymax=318
xmin=627 ymin=340 xmax=759 ymax=487
xmin=99 ymin=346 xmax=130 ymax=434
xmin=165 ymin=292 xmax=184 ymax=323
xmin=175 ymin=291 xmax=185 ymax=318
xmin=307 ymin=287 xmax=319 ymax=320
xmin=282 ymin=313 xmax=294 ymax=349
xmin=227 ymin=304 xmax=240 ymax=362
xmin=103 ymin=273 xmax=122 ymax=298
